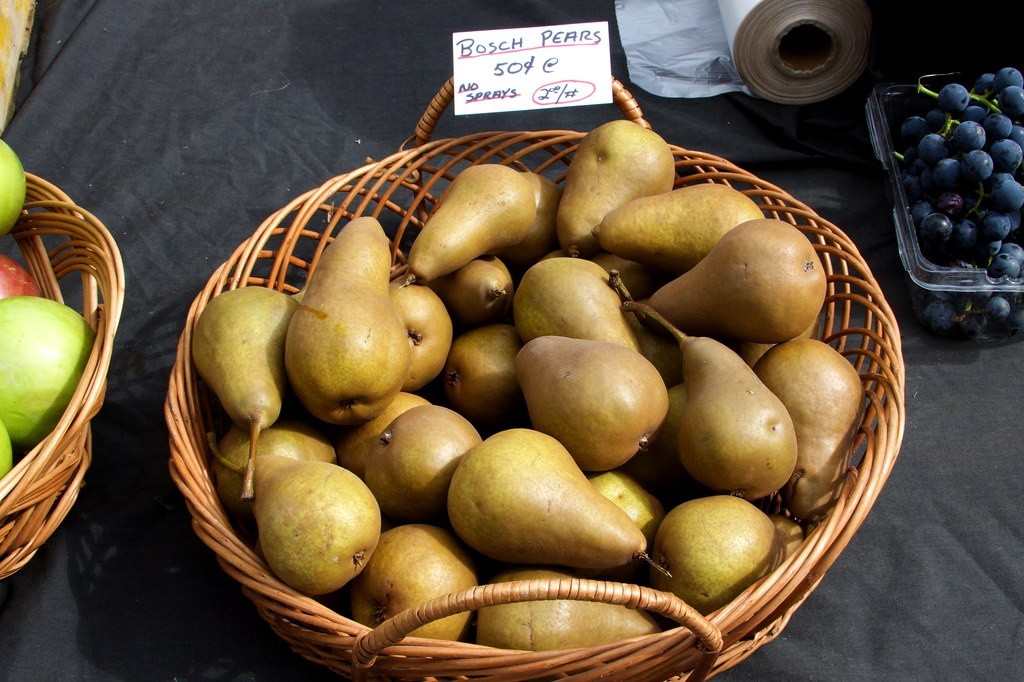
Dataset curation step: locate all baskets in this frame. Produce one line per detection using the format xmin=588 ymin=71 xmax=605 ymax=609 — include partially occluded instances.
xmin=166 ymin=75 xmax=906 ymax=682
xmin=0 ymin=171 xmax=127 ymax=580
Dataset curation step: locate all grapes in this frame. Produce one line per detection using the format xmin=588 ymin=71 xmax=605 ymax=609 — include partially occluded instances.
xmin=896 ymin=68 xmax=1024 ymax=339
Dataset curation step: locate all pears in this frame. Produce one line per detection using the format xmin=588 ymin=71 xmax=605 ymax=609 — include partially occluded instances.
xmin=191 ymin=119 xmax=865 ymax=650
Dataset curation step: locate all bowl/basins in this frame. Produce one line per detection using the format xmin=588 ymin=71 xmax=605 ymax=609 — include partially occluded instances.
xmin=864 ymin=81 xmax=1024 ymax=340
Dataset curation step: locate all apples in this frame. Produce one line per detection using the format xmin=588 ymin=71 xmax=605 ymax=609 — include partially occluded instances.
xmin=0 ymin=137 xmax=97 ymax=482
